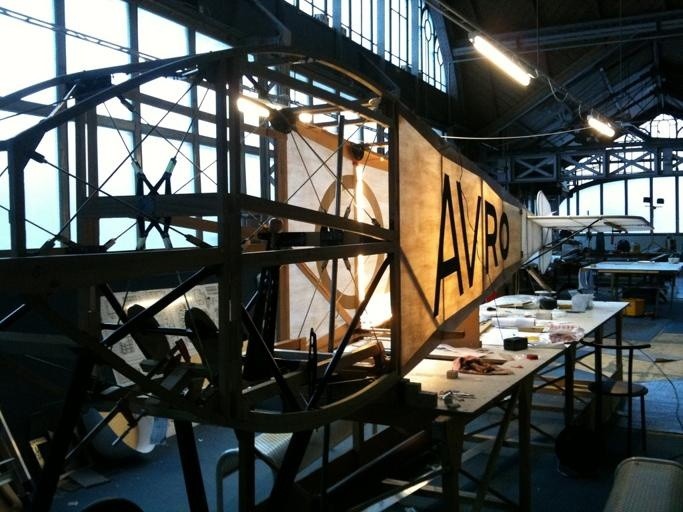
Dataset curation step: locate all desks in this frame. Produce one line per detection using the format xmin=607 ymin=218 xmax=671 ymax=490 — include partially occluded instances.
xmin=464 ymin=294 xmax=629 ymax=452
xmin=332 ymin=333 xmax=572 ymax=511
xmin=579 ymin=260 xmax=683 ymax=319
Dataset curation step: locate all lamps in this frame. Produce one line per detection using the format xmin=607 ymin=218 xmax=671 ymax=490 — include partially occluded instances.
xmin=469 ymin=33 xmax=532 ymax=87
xmin=584 ymin=113 xmax=638 ymax=141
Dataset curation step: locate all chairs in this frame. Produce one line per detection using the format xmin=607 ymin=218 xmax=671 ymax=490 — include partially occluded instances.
xmin=577 ymin=337 xmax=652 ymax=459
